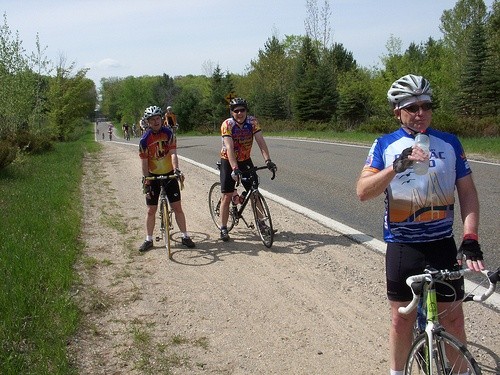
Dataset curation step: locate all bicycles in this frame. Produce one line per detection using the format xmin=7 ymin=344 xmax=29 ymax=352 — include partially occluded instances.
xmin=208 ymin=161 xmax=278 ymax=248
xmin=124 ymin=130 xmax=131 ymax=141
xmin=133 ymin=130 xmax=139 ymax=140
xmin=141 ymin=171 xmax=186 ymax=259
xmin=397 ymin=265 xmax=500 ymax=375
xmin=167 ymin=124 xmax=179 ymax=144
xmin=140 ymin=125 xmax=149 ymax=136
xmin=108 ymin=131 xmax=112 ymax=141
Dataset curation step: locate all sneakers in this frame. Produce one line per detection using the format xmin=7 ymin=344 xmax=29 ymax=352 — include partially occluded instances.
xmin=182 ymin=237 xmax=195 ymax=248
xmin=139 ymin=239 xmax=153 ymax=251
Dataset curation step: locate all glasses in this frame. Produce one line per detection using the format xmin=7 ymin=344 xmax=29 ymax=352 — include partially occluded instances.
xmin=232 ymin=108 xmax=246 ymax=114
xmin=404 ymin=102 xmax=432 ymax=113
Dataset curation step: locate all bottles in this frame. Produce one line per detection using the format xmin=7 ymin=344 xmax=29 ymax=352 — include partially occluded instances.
xmin=239 ymin=191 xmax=246 ymax=204
xmin=231 ymin=189 xmax=239 ymax=205
xmin=412 ymin=130 xmax=430 ymax=175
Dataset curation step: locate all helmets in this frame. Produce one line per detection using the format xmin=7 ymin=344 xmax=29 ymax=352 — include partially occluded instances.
xmin=229 ymin=97 xmax=247 ymax=106
xmin=387 ymin=74 xmax=434 ymax=103
xmin=143 ymin=105 xmax=163 ymax=120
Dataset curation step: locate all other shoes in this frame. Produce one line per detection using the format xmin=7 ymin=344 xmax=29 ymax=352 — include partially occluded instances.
xmin=221 ymin=229 xmax=229 ymax=241
xmin=260 ymin=225 xmax=275 ymax=235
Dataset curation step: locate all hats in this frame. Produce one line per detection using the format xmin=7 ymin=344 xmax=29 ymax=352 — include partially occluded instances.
xmin=167 ymin=106 xmax=172 ymax=110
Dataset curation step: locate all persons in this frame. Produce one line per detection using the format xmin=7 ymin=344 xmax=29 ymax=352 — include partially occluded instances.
xmin=356 ymin=74 xmax=487 ymax=375
xmin=220 ymin=98 xmax=277 ymax=241
xmin=95 ymin=106 xmax=176 ymax=141
xmin=138 ymin=105 xmax=195 ymax=252
